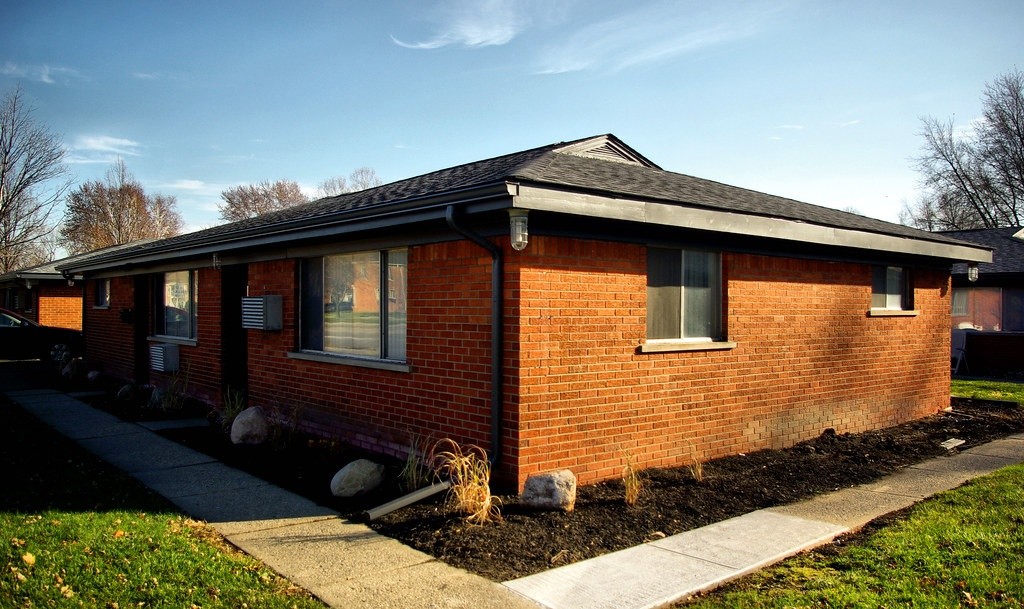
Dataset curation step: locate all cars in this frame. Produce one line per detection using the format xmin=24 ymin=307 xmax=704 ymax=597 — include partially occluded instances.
xmin=165 ymin=304 xmax=196 ymax=337
xmin=0 ymin=309 xmax=86 ymax=382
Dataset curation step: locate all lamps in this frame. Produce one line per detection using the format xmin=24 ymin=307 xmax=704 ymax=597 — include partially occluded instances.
xmin=509 ymin=209 xmax=528 ymax=251
xmin=968 ymin=262 xmax=979 ymax=282
xmin=67 ymin=274 xmax=74 ymax=286
xmin=213 ymin=252 xmax=223 ymax=270
xmin=26 ymin=279 xmax=32 ymax=289
